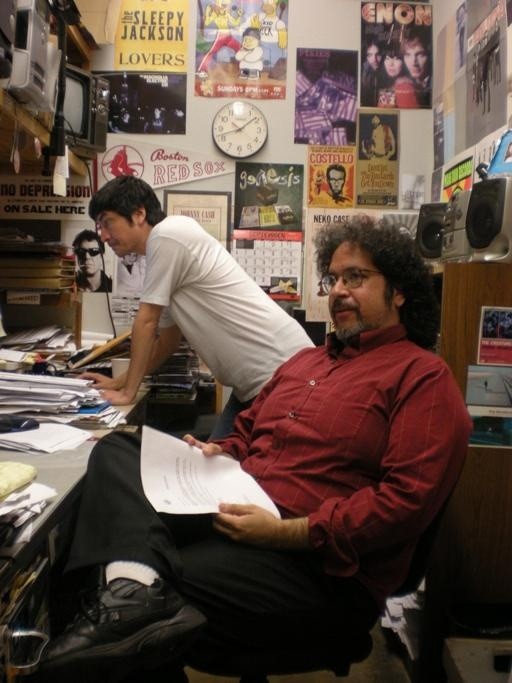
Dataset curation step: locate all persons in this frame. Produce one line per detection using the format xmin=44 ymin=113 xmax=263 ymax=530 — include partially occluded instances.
xmin=197 ymin=0 xmax=431 ymax=106
xmin=118 ymin=252 xmax=140 ymax=285
xmin=76 ymin=175 xmax=318 ymax=441
xmin=314 ymin=163 xmax=352 ymax=206
xmin=109 ymin=92 xmax=185 ymax=134
xmin=72 ymin=228 xmax=112 ymax=291
xmin=38 ymin=215 xmax=475 ymax=669
xmin=362 ymin=115 xmax=396 ymax=159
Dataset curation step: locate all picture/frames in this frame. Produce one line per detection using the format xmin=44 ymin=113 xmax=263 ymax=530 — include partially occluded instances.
xmin=161 ymin=185 xmax=233 ymax=258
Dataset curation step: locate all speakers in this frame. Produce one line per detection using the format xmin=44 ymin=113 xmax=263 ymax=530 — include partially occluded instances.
xmin=415 ymin=203 xmax=447 ymax=262
xmin=466 ymin=177 xmax=512 ymax=263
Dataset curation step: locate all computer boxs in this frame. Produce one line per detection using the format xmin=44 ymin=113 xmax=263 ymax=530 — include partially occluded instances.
xmin=0 ymin=0 xmax=51 ymax=105
xmin=0 ymin=0 xmax=18 ymax=78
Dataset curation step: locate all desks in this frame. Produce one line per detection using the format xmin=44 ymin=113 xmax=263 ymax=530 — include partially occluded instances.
xmin=1 ymin=367 xmax=151 ymax=682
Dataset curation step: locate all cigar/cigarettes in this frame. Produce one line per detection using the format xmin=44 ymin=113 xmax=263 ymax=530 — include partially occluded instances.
xmin=78 ymin=264 xmax=85 ymax=267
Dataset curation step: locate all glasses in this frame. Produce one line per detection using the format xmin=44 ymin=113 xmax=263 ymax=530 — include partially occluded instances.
xmin=318 ymin=267 xmax=383 ymax=294
xmin=73 ymin=248 xmax=100 ymax=256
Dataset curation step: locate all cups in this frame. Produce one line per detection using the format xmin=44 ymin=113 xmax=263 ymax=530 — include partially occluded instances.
xmin=109 ymin=358 xmax=131 ymax=379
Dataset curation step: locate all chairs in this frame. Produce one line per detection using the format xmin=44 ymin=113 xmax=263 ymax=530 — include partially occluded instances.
xmin=159 ymin=433 xmax=468 ymax=682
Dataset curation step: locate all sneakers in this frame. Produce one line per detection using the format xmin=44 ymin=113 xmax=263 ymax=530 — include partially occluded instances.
xmin=40 ymin=564 xmax=207 ymax=662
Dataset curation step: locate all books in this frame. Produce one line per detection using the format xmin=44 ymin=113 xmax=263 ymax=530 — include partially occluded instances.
xmin=0 ymin=255 xmax=77 ymax=289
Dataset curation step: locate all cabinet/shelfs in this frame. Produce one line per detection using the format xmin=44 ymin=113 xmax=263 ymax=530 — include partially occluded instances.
xmin=414 ymin=255 xmax=511 ymax=634
xmin=0 ymin=0 xmax=95 ymax=178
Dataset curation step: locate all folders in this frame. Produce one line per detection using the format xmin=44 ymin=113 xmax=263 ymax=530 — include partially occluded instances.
xmin=0 ymin=256 xmax=76 ymax=290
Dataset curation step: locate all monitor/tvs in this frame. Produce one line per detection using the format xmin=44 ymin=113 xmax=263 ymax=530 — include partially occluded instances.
xmin=63 ymin=62 xmax=111 ymax=160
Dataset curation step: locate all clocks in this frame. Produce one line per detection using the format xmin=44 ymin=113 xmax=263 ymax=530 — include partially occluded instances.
xmin=211 ymin=98 xmax=269 ymax=160
